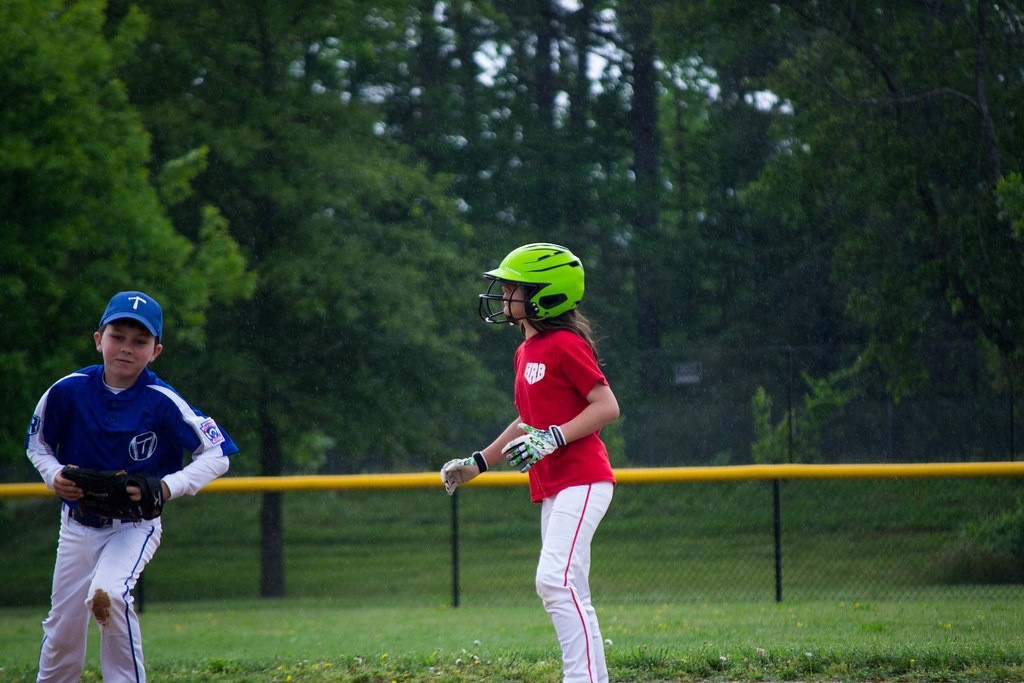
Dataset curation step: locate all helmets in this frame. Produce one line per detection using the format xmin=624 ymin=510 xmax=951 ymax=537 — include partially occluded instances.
xmin=482 ymin=243 xmax=585 ymax=320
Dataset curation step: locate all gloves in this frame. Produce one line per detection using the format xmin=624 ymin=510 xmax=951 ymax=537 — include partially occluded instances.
xmin=441 ymin=451 xmax=489 ymax=496
xmin=501 ymin=422 xmax=567 ymax=473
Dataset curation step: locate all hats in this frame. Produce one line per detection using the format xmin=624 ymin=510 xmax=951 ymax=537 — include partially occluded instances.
xmin=99 ymin=291 xmax=163 ymax=345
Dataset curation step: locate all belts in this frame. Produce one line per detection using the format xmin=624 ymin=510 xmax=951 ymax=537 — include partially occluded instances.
xmin=68 ymin=505 xmax=160 ymax=528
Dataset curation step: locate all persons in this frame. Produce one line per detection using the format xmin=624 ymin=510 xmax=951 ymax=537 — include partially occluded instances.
xmin=439 ymin=243 xmax=620 ymax=683
xmin=27 ymin=292 xmax=240 ymax=683
